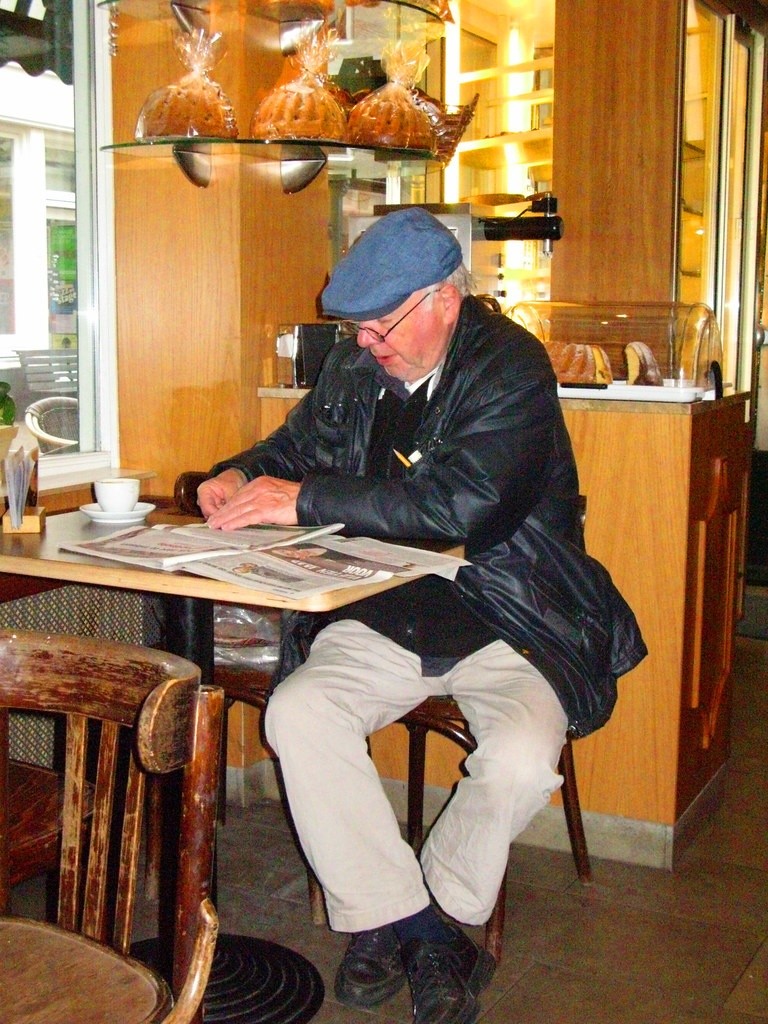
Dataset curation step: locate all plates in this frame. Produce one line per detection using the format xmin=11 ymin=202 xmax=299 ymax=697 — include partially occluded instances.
xmin=79 ymin=503 xmax=156 ymax=523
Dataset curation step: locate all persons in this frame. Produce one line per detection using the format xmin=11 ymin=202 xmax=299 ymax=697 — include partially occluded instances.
xmin=196 ymin=206 xmax=648 ymax=1024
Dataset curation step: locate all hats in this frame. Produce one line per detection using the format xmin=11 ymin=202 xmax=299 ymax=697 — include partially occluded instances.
xmin=321 ymin=207 xmax=463 ymax=322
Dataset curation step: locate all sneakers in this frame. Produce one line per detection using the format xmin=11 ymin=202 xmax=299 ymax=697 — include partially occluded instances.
xmin=398 ymin=922 xmax=497 ymax=1024
xmin=333 ymin=924 xmax=408 ymax=1010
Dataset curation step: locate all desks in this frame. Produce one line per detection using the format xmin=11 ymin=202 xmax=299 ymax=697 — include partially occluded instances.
xmin=0 ymin=508 xmax=468 ymax=1024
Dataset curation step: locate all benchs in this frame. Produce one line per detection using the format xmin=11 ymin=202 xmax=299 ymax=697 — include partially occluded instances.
xmin=13 ymin=349 xmax=78 ymax=398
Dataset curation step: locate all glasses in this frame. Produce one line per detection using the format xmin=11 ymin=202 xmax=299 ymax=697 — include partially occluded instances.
xmin=338 ymin=289 xmax=441 ymax=343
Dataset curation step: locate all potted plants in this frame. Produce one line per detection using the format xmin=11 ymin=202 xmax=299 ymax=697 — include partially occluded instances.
xmin=0 ymin=381 xmax=19 ymax=462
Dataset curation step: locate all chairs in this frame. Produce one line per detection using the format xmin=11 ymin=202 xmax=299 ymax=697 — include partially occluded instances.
xmin=3 ymin=756 xmax=99 ymax=927
xmin=24 ymin=397 xmax=78 ymax=454
xmin=214 ymin=603 xmax=331 ymax=926
xmin=371 ymin=494 xmax=595 ymax=966
xmin=0 ymin=630 xmax=227 ymax=1024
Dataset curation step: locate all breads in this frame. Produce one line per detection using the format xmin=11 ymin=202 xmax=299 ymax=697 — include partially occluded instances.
xmin=350 ymin=102 xmax=435 ymax=148
xmin=625 ymin=342 xmax=664 ymax=386
xmin=253 ymin=91 xmax=346 ymax=141
xmin=144 ymin=90 xmax=230 ymax=138
xmin=543 ymin=342 xmax=614 ymax=384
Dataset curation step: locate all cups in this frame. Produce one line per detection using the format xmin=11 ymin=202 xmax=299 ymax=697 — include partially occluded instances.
xmin=94 ymin=478 xmax=141 ymax=512
